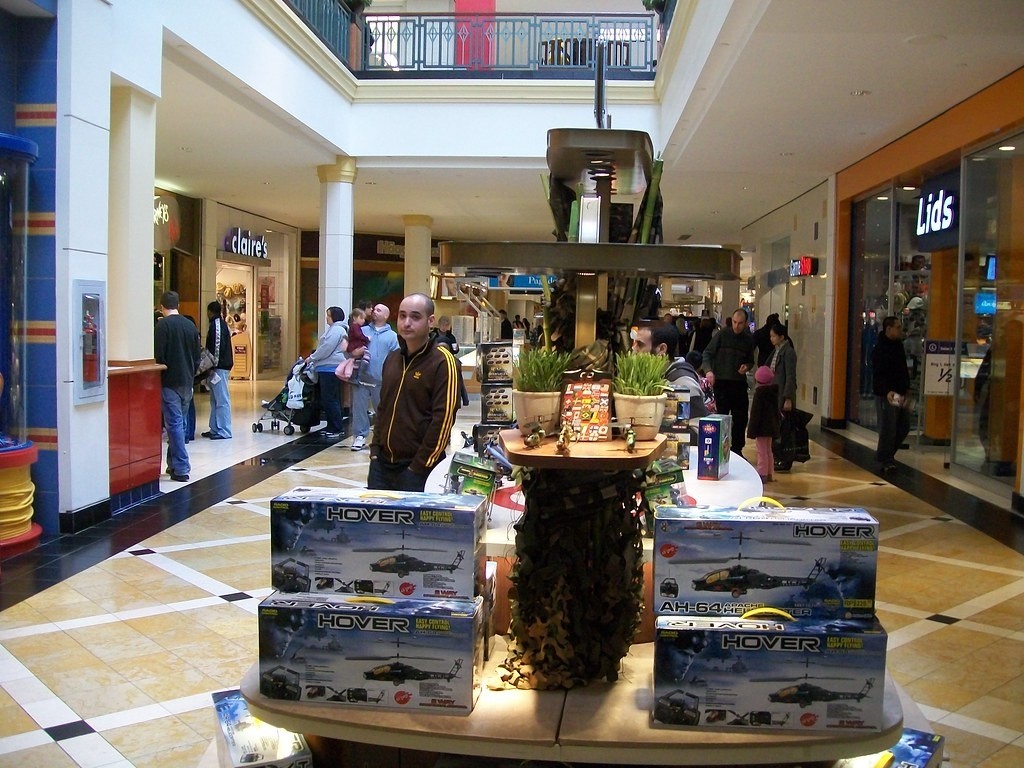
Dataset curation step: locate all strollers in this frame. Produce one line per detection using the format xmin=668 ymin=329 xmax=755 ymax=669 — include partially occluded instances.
xmin=252 ymin=359 xmax=322 ymax=436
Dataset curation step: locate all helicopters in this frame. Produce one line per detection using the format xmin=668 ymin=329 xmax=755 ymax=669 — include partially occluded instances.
xmin=345 ymin=652 xmax=464 ymax=686
xmin=749 ymin=673 xmax=875 ymax=709
xmin=352 ymin=545 xmax=466 ymax=578
xmin=668 ymin=552 xmax=826 ymax=598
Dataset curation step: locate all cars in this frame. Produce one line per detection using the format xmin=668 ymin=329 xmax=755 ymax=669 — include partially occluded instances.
xmin=654 ymin=689 xmax=701 ymax=726
xmin=660 ymin=577 xmax=679 ymax=598
xmin=261 ymin=666 xmax=302 ymax=701
xmin=273 ymin=558 xmax=311 ymax=594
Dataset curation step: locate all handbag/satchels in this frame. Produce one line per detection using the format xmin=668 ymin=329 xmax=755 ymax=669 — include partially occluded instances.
xmin=194 ymin=344 xmax=218 ymax=385
xmin=286 ymin=374 xmax=305 ymax=409
xmin=779 ymin=408 xmax=814 ymax=463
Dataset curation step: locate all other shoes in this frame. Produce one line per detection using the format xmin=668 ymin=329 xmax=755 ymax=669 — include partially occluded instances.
xmin=166 ymin=467 xmax=189 ymax=482
xmin=875 ymin=457 xmax=898 ymax=471
xmin=367 ymin=410 xmax=373 ymax=415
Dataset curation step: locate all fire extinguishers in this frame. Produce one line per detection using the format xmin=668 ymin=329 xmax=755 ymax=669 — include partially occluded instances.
xmin=82 ymin=311 xmax=99 ymax=382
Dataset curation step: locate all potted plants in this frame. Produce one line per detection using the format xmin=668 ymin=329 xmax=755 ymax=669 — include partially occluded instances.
xmin=503 ymin=346 xmax=572 ymax=440
xmin=610 ymin=347 xmax=678 ymax=442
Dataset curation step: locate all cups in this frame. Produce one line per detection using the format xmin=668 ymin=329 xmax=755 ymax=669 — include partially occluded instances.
xmin=451 ymin=315 xmax=473 ymax=344
xmin=891 ymin=394 xmax=900 ymax=407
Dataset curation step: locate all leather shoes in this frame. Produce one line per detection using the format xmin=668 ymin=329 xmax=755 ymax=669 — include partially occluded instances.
xmin=201 ymin=432 xmax=232 ymax=440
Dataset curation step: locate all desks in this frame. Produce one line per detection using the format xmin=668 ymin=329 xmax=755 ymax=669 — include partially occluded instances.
xmin=498 ymin=427 xmax=671 ymax=688
xmin=425 ymin=446 xmax=765 ymax=555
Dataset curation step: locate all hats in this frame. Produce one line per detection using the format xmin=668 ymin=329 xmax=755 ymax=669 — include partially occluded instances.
xmin=207 ymin=300 xmax=221 ymax=313
xmin=885 ymin=282 xmax=906 ymax=313
xmin=755 ymin=366 xmax=774 ymax=383
xmin=907 ymin=297 xmax=924 ymax=309
xmin=160 ymin=291 xmax=179 ymax=308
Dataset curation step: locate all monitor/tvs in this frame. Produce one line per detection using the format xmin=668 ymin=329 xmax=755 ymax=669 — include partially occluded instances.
xmin=985 ymin=255 xmax=996 ymax=280
xmin=972 ymin=292 xmax=997 ymax=315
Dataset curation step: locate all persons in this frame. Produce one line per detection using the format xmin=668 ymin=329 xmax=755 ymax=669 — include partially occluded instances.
xmin=748 ymin=366 xmax=781 ymax=483
xmin=769 ymin=322 xmax=799 ymax=471
xmin=499 ymin=309 xmax=513 ymax=339
xmin=628 ymin=322 xmax=711 ymax=447
xmin=974 ymin=319 xmax=1023 ymax=478
xmin=865 ymin=309 xmax=879 ymax=371
xmin=305 ymin=308 xmax=348 ymax=441
xmin=202 ymin=302 xmax=234 ymax=441
xmin=753 ymin=312 xmax=795 ymax=368
xmin=703 ymin=308 xmax=754 ymax=462
xmin=430 ymin=314 xmax=469 ymax=445
xmin=870 ymin=315 xmax=911 ymax=467
xmin=344 ymin=304 xmax=402 ymax=450
xmin=367 ymin=294 xmax=457 ymax=493
xmin=664 ymin=312 xmax=733 ymax=359
xmin=154 ymin=290 xmax=202 ymax=484
xmin=898 ymin=307 xmax=911 ymax=338
xmin=513 ymin=314 xmax=530 ymax=339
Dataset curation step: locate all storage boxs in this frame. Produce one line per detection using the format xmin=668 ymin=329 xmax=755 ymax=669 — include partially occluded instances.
xmin=677 ymin=430 xmax=690 ymax=470
xmin=642 ymin=485 xmax=678 ymax=536
xmin=210 ymin=687 xmax=313 ymax=768
xmin=475 ymin=342 xmax=513 ymax=383
xmin=267 ymin=486 xmax=490 ymax=599
xmin=698 ymin=412 xmax=736 ymax=481
xmin=471 ymin=422 xmax=504 ymax=457
xmin=645 ymin=457 xmax=686 ymax=488
xmin=836 ymin=727 xmax=947 ymax=768
xmin=652 ymin=610 xmax=901 ymax=731
xmin=649 ymin=502 xmax=882 ymax=615
xmin=480 ymin=383 xmax=516 ymax=425
xmin=481 ymin=561 xmax=504 ymax=656
xmin=441 ymin=448 xmax=499 ymax=496
xmin=559 ymin=380 xmax=610 ymax=441
xmin=261 ymin=591 xmax=488 ymax=718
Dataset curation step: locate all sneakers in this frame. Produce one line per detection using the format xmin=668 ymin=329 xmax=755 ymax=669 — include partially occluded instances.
xmin=351 ymin=435 xmax=366 ymax=451
xmin=760 ymin=474 xmax=773 ymax=484
xmin=320 ymin=431 xmax=345 ymax=439
